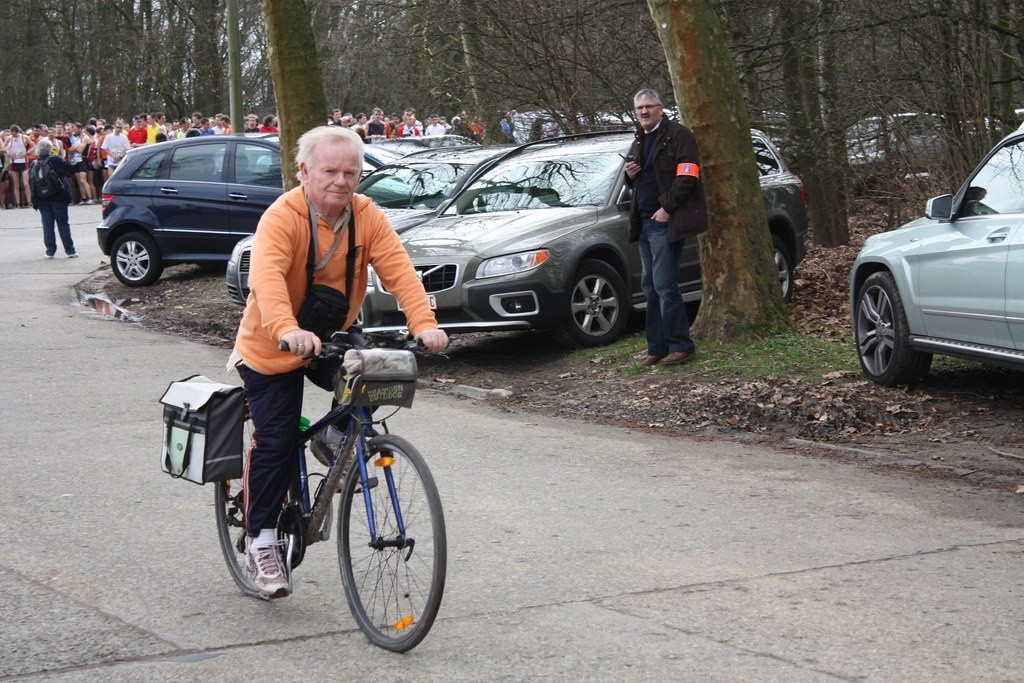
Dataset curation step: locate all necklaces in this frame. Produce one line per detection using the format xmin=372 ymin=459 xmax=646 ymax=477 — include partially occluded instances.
xmin=305 ymin=193 xmax=351 ymax=271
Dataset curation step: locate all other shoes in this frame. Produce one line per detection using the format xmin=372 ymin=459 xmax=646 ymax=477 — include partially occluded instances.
xmin=68 ymin=198 xmax=102 ymax=205
xmin=0 ymin=202 xmax=33 ymax=209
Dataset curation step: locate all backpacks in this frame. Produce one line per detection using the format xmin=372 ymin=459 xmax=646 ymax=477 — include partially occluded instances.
xmin=33 ymin=154 xmax=64 ymax=201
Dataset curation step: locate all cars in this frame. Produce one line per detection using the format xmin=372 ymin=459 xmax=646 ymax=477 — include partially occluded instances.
xmin=847 ymin=110 xmax=952 ymax=171
xmin=373 ymin=134 xmax=483 ymax=156
xmin=223 ymin=141 xmax=561 ymax=313
xmin=93 ymin=130 xmax=386 ymax=288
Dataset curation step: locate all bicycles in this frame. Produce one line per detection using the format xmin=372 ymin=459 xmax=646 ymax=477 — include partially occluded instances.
xmin=210 ymin=330 xmax=455 ymax=655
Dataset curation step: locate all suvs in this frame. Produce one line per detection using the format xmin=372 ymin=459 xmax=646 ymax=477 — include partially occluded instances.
xmin=349 ymin=120 xmax=811 ymax=349
xmin=845 ymin=125 xmax=1024 ymax=389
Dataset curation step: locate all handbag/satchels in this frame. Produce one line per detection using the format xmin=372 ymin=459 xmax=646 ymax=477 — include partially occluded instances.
xmin=159 ymin=375 xmax=246 ymax=486
xmin=295 ymin=285 xmax=349 ymax=342
xmin=333 ymin=347 xmax=417 ymax=408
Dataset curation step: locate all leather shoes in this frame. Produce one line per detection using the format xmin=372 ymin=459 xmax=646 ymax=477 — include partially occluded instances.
xmin=660 ymin=352 xmax=695 ymax=364
xmin=640 ymin=355 xmax=664 ymax=364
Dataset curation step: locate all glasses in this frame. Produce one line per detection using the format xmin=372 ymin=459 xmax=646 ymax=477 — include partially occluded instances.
xmin=115 ymin=126 xmax=122 ymax=129
xmin=636 ymin=103 xmax=661 ymax=109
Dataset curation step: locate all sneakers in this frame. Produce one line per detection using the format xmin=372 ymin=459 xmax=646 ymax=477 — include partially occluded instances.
xmin=245 ymin=539 xmax=293 ymax=600
xmin=44 ymin=253 xmax=53 ymax=259
xmin=310 ymin=428 xmax=364 ymax=494
xmin=69 ymin=252 xmax=79 ymax=258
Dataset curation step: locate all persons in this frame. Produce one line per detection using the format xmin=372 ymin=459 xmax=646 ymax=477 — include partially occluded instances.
xmin=624 ymin=88 xmax=710 ymax=368
xmin=28 ymin=138 xmax=80 ymax=258
xmin=0 ymin=110 xmax=131 ymax=212
xmin=131 ymin=100 xmax=484 ymax=149
xmin=223 ymin=124 xmax=448 ymax=598
